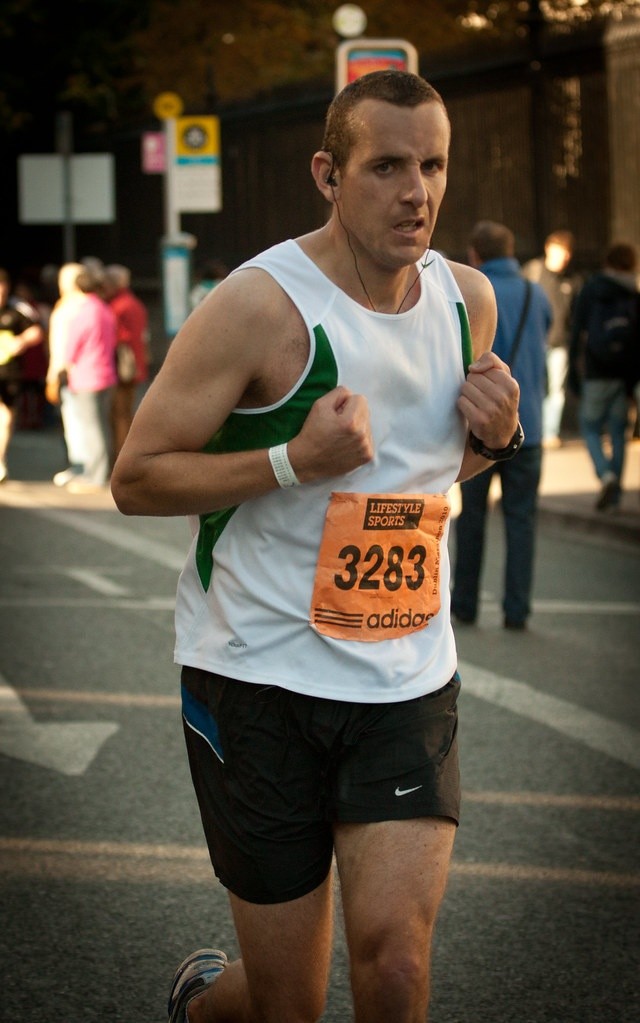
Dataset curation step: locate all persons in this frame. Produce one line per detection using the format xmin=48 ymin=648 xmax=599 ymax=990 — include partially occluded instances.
xmin=101 ymin=266 xmax=149 ymax=480
xmin=46 ymin=260 xmax=118 ymax=495
xmin=0 ymin=271 xmax=52 ymax=486
xmin=564 ymin=242 xmax=640 ymax=513
xmin=108 ymin=72 xmax=523 ymax=1023
xmin=522 ymin=229 xmax=583 ymax=452
xmin=449 ymin=218 xmax=554 ymax=634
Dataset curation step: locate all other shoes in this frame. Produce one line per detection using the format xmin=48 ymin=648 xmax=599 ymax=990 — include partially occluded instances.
xmin=505 ymin=617 xmax=524 ymax=629
xmin=53 ymin=467 xmax=108 ymax=494
xmin=451 ymin=605 xmax=476 ymax=622
xmin=595 ymin=476 xmax=620 ymax=513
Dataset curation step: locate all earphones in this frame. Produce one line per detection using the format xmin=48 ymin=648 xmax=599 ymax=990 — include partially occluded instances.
xmin=325 ymin=173 xmax=337 ymax=187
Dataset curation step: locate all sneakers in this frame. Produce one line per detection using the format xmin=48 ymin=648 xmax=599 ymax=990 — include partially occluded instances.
xmin=168 ymin=948 xmax=231 ymax=1023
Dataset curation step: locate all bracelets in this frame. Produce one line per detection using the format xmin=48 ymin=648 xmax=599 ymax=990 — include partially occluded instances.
xmin=267 ymin=441 xmax=302 ymax=491
xmin=469 ymin=421 xmax=526 ymax=461
